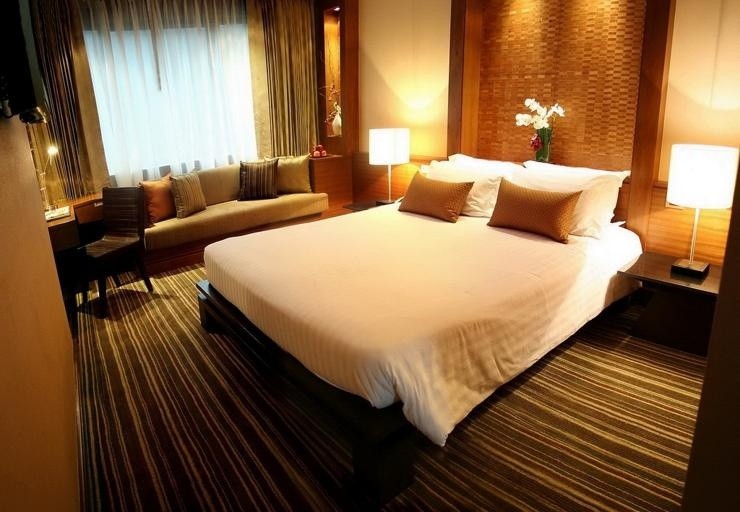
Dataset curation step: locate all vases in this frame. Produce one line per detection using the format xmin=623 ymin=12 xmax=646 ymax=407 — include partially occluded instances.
xmin=331 ymin=113 xmax=342 ymax=136
xmin=535 ymin=128 xmax=552 ymax=163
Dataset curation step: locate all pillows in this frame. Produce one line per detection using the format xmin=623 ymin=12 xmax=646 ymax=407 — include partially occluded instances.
xmin=513 ymin=170 xmax=621 ymax=241
xmin=522 ymin=160 xmax=633 ymax=235
xmin=424 ymin=159 xmax=508 ymax=219
xmin=263 ymin=152 xmax=314 ymax=194
xmin=137 ymin=172 xmax=182 ymax=222
xmin=397 ymin=170 xmax=476 ymax=224
xmin=486 ymin=178 xmax=584 ymax=246
xmin=448 ymin=152 xmax=526 ymax=171
xmin=168 ymin=168 xmax=207 ymax=220
xmin=237 ymin=157 xmax=280 ymax=202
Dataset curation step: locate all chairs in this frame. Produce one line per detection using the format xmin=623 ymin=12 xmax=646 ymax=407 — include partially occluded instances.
xmin=75 ymin=186 xmax=154 ymax=320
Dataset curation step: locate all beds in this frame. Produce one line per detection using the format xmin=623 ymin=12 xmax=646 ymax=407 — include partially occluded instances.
xmin=194 ymin=152 xmax=644 ymax=450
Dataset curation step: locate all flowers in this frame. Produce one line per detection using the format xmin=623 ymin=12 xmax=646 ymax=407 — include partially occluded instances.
xmin=514 ymin=97 xmax=566 ymax=152
xmin=313 ymin=41 xmax=342 ymax=125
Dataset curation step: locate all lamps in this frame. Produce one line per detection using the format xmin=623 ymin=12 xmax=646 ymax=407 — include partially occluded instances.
xmin=368 ymin=128 xmax=411 ymax=207
xmin=665 ymin=143 xmax=739 ymax=276
xmin=39 ymin=144 xmax=60 ymax=211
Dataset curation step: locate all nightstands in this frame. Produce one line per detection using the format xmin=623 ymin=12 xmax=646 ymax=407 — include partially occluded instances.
xmin=342 ymin=202 xmax=377 ymax=211
xmin=617 ymin=251 xmax=723 ymax=358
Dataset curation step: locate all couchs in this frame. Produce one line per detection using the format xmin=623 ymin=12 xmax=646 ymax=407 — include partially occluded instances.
xmin=137 ymin=163 xmax=327 ymax=266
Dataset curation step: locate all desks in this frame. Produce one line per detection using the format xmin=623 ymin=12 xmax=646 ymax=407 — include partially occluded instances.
xmin=43 ymin=191 xmax=104 ymax=338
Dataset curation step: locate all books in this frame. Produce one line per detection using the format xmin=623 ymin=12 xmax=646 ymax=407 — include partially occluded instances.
xmin=45 ymin=206 xmax=70 ymax=221
